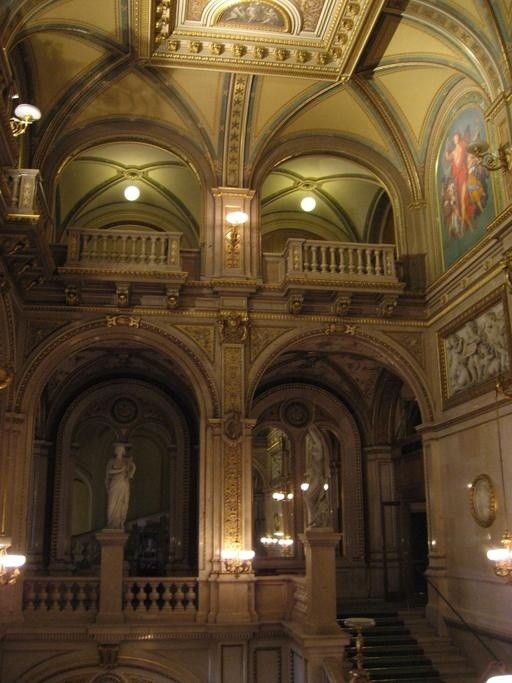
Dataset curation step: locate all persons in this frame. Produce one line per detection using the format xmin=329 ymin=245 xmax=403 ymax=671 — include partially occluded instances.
xmin=443 ymin=133 xmax=487 ymax=241
xmin=444 ymin=312 xmax=510 ymax=398
xmin=226 ymin=2 xmax=277 ymax=25
xmin=104 ymin=446 xmax=137 ymax=529
xmin=302 ymin=425 xmax=331 ymax=528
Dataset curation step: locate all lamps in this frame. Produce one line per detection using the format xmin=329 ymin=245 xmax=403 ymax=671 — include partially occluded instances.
xmin=9 ymin=103 xmax=43 ymax=139
xmin=0 ymin=535 xmax=27 ymax=588
xmin=486 ymin=528 xmax=512 ymax=581
xmin=124 ymin=171 xmax=141 ymax=202
xmin=219 ymin=544 xmax=256 ymax=581
xmin=299 ymin=180 xmax=318 ymax=213
xmin=464 ymin=138 xmax=511 ymax=174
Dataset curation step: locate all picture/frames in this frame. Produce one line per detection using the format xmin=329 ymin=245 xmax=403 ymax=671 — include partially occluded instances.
xmin=469 ymin=474 xmax=497 ymax=530
